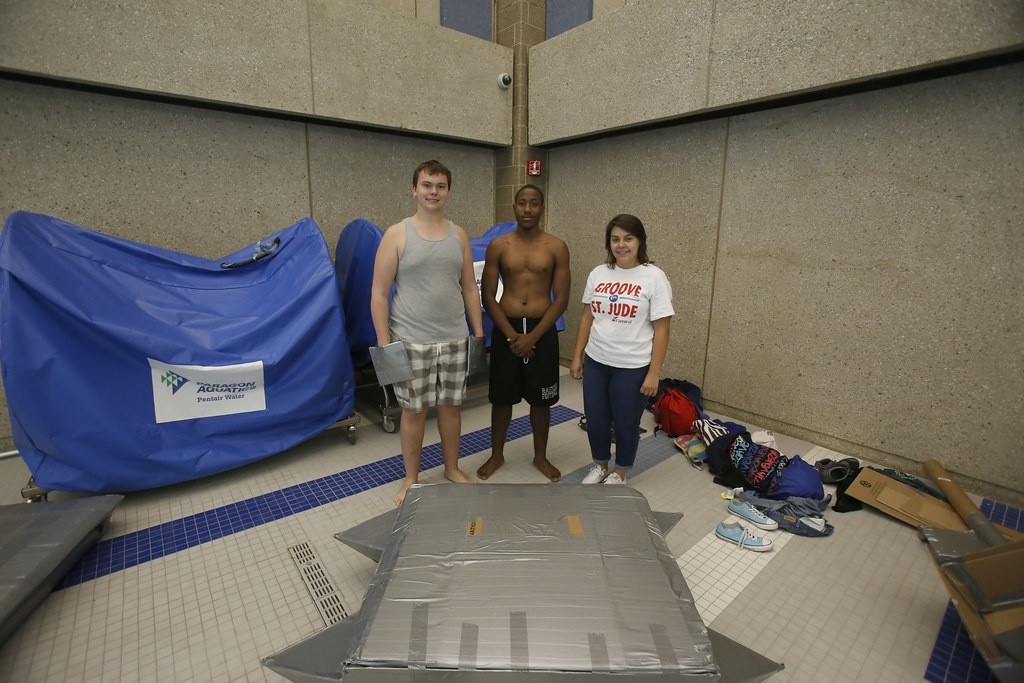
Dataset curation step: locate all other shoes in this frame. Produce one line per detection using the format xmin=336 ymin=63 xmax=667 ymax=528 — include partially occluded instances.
xmin=814 ymin=458 xmax=860 ymax=484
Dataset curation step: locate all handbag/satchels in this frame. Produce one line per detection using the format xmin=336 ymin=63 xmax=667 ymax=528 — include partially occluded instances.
xmin=649 ymin=378 xmax=703 ymax=413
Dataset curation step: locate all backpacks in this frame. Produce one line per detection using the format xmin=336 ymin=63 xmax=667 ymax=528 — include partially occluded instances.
xmin=653 ymin=386 xmax=701 ymax=438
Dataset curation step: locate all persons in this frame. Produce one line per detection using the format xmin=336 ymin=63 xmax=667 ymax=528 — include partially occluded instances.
xmin=569 ymin=214 xmax=675 ymax=485
xmin=477 ymin=185 xmax=571 ymax=482
xmin=371 ymin=160 xmax=483 ymax=509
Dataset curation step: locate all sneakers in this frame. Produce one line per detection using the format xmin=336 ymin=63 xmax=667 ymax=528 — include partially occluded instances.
xmin=727 ymin=498 xmax=779 ymax=531
xmin=715 ymin=520 xmax=774 ymax=552
xmin=580 ymin=463 xmax=609 ymax=484
xmin=601 ymin=471 xmax=627 ymax=484
